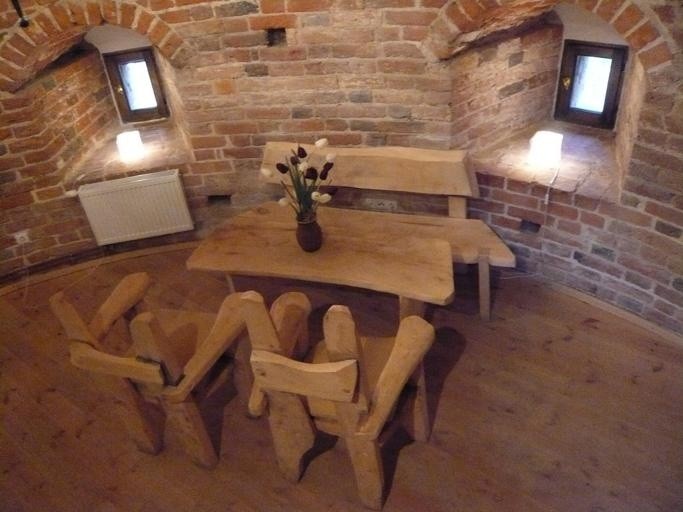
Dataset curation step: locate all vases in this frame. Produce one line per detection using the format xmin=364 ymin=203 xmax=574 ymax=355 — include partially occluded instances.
xmin=291 ymin=213 xmax=325 ymax=253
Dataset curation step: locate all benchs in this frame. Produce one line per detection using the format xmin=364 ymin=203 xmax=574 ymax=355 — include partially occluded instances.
xmin=253 ymin=136 xmax=520 ymax=325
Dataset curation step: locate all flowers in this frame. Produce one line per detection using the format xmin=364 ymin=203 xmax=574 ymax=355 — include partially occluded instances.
xmin=256 ymin=130 xmax=339 ymax=220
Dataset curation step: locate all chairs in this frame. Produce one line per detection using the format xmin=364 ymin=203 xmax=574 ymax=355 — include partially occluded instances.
xmin=236 ymin=287 xmax=437 ymax=510
xmin=44 ymin=268 xmax=253 ymax=476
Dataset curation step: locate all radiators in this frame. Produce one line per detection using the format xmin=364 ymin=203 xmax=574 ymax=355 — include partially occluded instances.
xmin=72 ymin=167 xmax=196 ymax=247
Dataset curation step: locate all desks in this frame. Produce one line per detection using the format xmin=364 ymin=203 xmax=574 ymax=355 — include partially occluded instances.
xmin=179 ymin=198 xmax=458 ymax=337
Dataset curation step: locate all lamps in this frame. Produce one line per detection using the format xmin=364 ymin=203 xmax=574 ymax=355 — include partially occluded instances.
xmin=522 ymin=122 xmax=566 ymax=180
xmin=111 ymin=124 xmax=145 ymax=164
